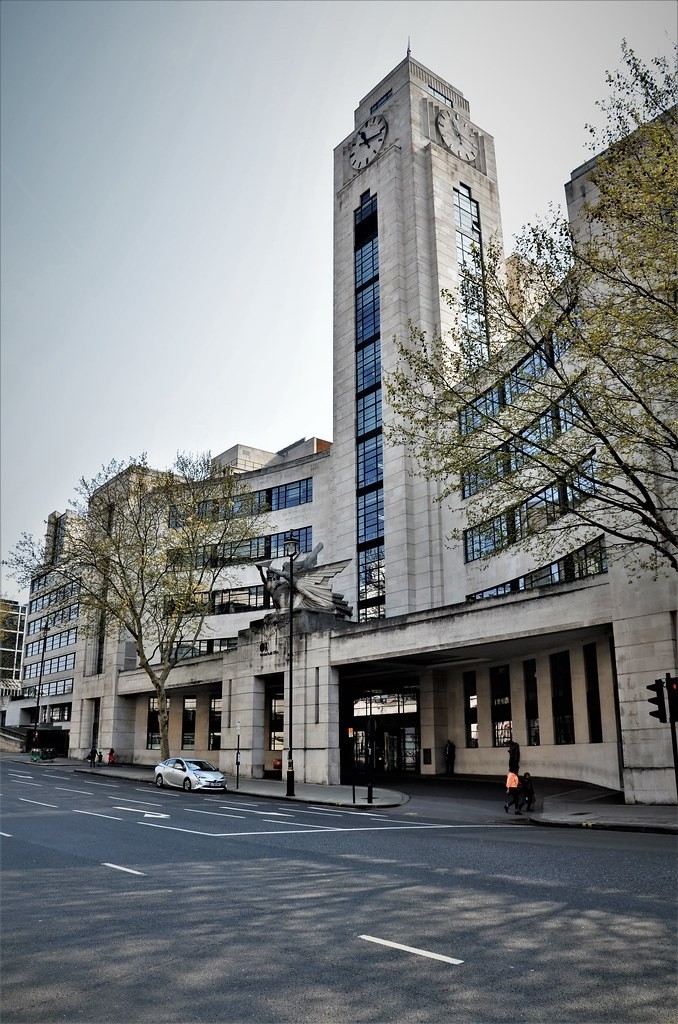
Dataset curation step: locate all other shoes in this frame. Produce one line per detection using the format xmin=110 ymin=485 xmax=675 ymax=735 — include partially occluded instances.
xmin=515 ymin=810 xmax=522 ymax=815
xmin=526 ymin=808 xmax=533 ymax=811
xmin=504 ymin=805 xmax=509 ymax=813
xmin=519 ymin=804 xmax=521 ymax=811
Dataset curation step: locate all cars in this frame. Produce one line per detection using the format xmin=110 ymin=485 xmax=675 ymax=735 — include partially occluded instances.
xmin=155 ymin=757 xmax=228 ymax=795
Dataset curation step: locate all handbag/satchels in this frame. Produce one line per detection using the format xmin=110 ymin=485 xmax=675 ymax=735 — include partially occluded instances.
xmin=518 ymin=784 xmax=522 ymax=793
xmin=532 ymin=795 xmax=536 ymax=803
xmin=86 ymin=752 xmax=92 ymax=759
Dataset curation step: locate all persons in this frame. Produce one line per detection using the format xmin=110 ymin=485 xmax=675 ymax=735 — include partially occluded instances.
xmin=90 ymin=746 xmax=97 ymax=767
xmin=506 ymin=741 xmax=520 ymax=773
xmin=97 ymin=751 xmax=103 ymax=766
xmin=445 ymin=740 xmax=455 ymax=774
xmin=255 ymin=558 xmax=352 ymax=608
xmin=108 ymin=748 xmax=115 ymax=763
xmin=519 ymin=772 xmax=535 ymax=811
xmin=505 ymin=765 xmax=522 ymax=815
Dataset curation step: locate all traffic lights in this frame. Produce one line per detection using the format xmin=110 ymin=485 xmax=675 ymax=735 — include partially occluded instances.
xmin=671 ymin=677 xmax=678 ymax=722
xmin=645 ymin=679 xmax=666 ymax=723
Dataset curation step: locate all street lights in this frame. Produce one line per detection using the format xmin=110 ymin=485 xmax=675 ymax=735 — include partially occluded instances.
xmin=282 ymin=527 xmax=300 ymax=798
xmin=32 ymin=621 xmax=51 ymax=748
